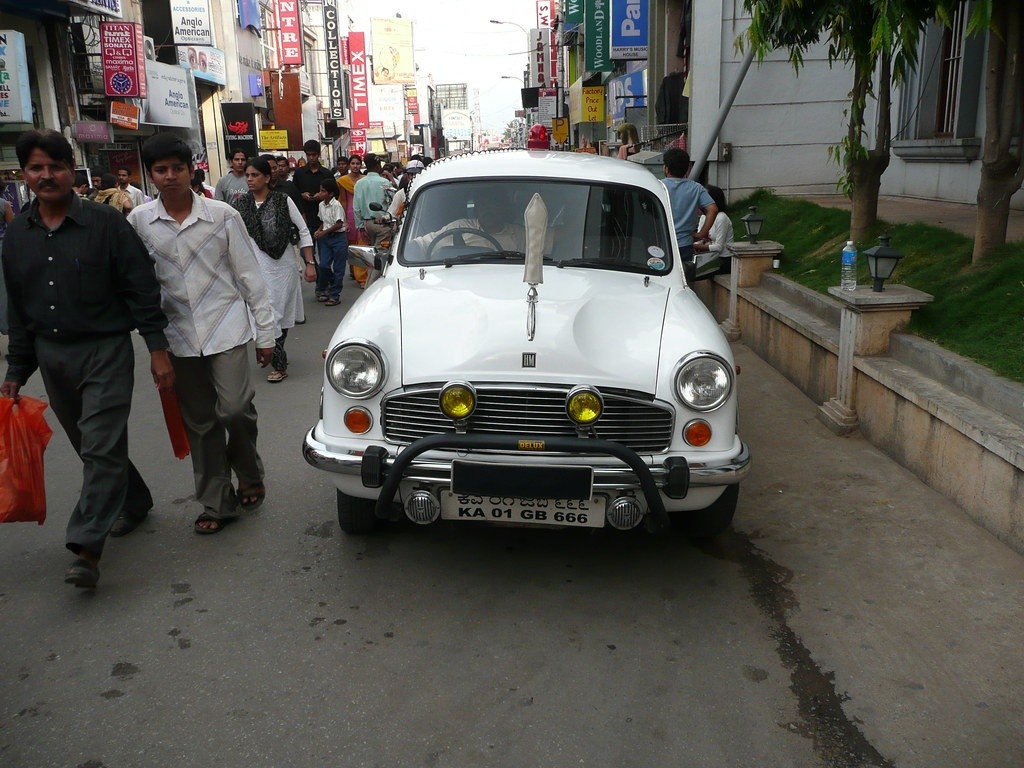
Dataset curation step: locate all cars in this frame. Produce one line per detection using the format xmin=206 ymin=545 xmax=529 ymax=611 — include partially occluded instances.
xmin=300 ymin=148 xmax=752 ymax=550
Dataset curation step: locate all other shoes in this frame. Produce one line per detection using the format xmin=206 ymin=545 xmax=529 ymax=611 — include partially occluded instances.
xmin=64 ymin=557 xmax=100 ymax=589
xmin=110 ymin=509 xmax=148 ymax=538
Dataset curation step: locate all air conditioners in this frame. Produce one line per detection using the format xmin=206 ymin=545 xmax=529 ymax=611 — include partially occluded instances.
xmin=143 ymin=35 xmax=156 ymax=62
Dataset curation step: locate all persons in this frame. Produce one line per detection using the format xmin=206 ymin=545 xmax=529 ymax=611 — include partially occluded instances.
xmin=216 ymin=140 xmax=434 ymax=382
xmin=0 ymin=181 xmax=15 ymax=333
xmin=125 ymin=134 xmax=277 ymax=535
xmin=0 ymin=125 xmax=177 ymax=590
xmin=190 ymin=168 xmax=215 ymax=199
xmin=658 ymin=148 xmax=718 ymax=290
xmin=616 ymin=123 xmax=641 ymax=160
xmin=404 ymin=183 xmax=554 ymax=261
xmin=73 ymin=166 xmax=152 ymax=217
xmin=693 ymin=184 xmax=734 ymax=281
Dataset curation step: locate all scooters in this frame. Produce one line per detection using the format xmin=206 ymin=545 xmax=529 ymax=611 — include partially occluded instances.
xmin=348 ymin=201 xmax=399 ymax=292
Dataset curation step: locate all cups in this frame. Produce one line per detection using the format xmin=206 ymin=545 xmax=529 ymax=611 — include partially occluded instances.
xmin=773 ymin=260 xmax=780 ymax=268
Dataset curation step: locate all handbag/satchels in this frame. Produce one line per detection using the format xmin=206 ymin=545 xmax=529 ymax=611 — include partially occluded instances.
xmin=0 ymin=394 xmax=52 ymax=525
xmin=158 ymin=381 xmax=191 ymax=460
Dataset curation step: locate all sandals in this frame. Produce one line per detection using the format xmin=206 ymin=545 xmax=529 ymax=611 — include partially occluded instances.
xmin=237 ymin=479 xmax=265 ymax=511
xmin=195 ymin=511 xmax=239 ymax=534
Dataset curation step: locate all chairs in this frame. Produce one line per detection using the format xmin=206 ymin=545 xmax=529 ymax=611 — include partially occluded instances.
xmin=511 ymin=186 xmax=544 ymax=229
xmin=553 ymin=201 xmax=626 ymax=261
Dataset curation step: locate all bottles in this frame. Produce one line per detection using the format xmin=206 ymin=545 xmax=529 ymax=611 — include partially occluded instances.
xmin=841 ymin=241 xmax=857 ymax=292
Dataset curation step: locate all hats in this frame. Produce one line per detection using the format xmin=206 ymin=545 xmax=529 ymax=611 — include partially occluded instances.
xmin=406 ymin=160 xmax=424 ymax=173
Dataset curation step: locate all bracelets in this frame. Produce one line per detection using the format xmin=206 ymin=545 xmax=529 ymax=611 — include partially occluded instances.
xmin=306 ymin=261 xmax=315 ymax=265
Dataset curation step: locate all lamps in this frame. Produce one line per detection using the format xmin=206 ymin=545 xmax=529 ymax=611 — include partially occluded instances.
xmin=862 ymin=229 xmax=905 ymax=293
xmin=739 ymin=206 xmax=766 ymax=245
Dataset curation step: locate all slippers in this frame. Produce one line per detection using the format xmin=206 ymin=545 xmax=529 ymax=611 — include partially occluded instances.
xmin=324 ymin=300 xmax=341 ymax=306
xmin=267 ymin=369 xmax=288 ymax=382
xmin=317 ymin=295 xmax=329 ymax=301
